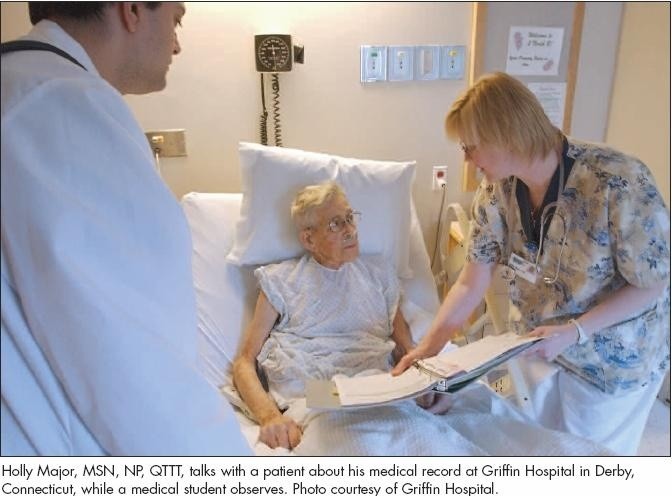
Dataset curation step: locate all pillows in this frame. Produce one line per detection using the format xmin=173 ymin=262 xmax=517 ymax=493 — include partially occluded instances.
xmin=225 ymin=141 xmax=417 ymax=279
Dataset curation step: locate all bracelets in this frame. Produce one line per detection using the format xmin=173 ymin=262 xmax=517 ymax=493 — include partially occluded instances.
xmin=569 ymin=318 xmax=590 ymax=347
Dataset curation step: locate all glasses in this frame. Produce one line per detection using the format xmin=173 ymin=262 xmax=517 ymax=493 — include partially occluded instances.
xmin=304 ymin=211 xmax=362 ymax=233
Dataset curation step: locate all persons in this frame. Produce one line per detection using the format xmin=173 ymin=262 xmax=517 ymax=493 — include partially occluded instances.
xmin=2 ymin=2 xmax=254 ymax=456
xmin=390 ymin=71 xmax=669 ymax=455
xmin=229 ymin=178 xmax=456 ymax=452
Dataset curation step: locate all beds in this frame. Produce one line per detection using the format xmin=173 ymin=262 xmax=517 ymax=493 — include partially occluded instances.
xmin=182 ymin=192 xmax=621 ymax=456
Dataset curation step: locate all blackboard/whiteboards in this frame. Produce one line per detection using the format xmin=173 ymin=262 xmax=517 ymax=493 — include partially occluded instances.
xmin=464 ymin=2 xmax=625 ymax=191
